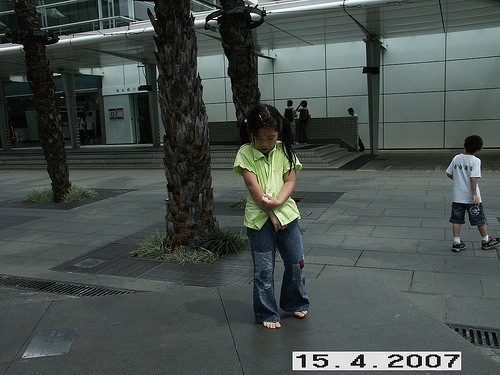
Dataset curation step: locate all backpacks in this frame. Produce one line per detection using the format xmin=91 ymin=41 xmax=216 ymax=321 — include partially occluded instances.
xmin=285 ymin=108 xmax=293 ymax=122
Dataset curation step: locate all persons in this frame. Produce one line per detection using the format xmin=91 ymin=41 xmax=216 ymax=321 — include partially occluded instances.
xmin=284 ymin=100 xmax=298 ymax=145
xmin=445 ymin=135 xmax=500 ymax=252
xmin=296 ymin=100 xmax=311 ymax=145
xmin=9 ymin=120 xmax=17 ymax=147
xmin=347 ymin=108 xmax=365 ymax=152
xmin=77 ymin=113 xmax=86 ymax=145
xmin=232 ymin=105 xmax=310 ymax=328
xmin=85 ymin=112 xmax=96 ymax=145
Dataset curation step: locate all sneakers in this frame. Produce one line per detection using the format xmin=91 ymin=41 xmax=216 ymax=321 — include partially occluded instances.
xmin=452 ymin=241 xmax=466 ymax=252
xmin=481 ymin=237 xmax=500 ymax=250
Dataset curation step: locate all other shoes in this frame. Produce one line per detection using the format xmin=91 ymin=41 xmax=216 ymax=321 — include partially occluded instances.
xmin=295 ymin=142 xmax=300 ymax=145
